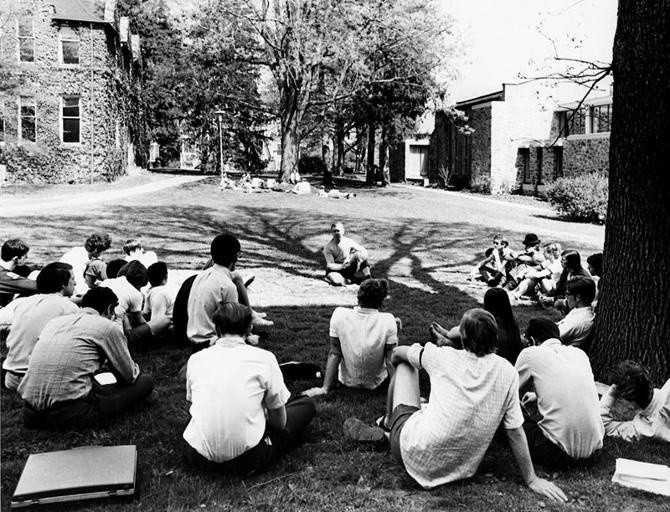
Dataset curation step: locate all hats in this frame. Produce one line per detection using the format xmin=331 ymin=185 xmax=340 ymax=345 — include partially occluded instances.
xmin=523 ymin=234 xmax=539 ymax=244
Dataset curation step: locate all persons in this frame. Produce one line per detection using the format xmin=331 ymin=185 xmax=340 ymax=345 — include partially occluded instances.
xmin=319 ymin=223 xmax=373 ymax=285
xmin=512 ymin=317 xmax=607 ymax=465
xmin=425 ymin=289 xmax=523 ymax=368
xmin=597 ymin=357 xmax=670 ymax=443
xmin=173 ymin=304 xmax=318 ymax=482
xmin=342 ymin=311 xmax=571 ymax=505
xmin=221 ymin=158 xmax=357 ymax=200
xmin=469 ymin=234 xmax=611 ymax=346
xmin=299 ymin=278 xmax=405 ymax=399
xmin=0 ymin=232 xmax=277 ymax=432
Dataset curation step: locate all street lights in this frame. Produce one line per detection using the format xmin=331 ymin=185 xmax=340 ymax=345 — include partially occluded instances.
xmin=215 ymin=108 xmax=227 ymax=184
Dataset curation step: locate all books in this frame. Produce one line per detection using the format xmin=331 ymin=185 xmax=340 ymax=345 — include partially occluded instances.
xmin=610 ymin=457 xmax=670 ymax=498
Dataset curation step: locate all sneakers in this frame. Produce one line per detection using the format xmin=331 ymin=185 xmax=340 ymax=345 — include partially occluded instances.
xmin=343 ymin=418 xmax=384 ymax=441
